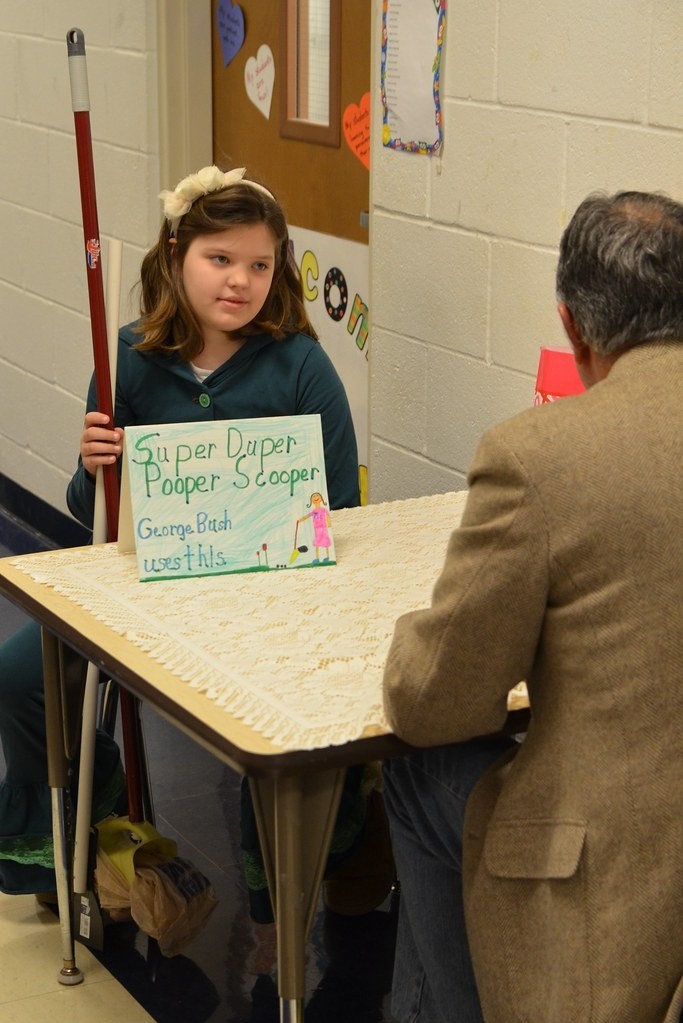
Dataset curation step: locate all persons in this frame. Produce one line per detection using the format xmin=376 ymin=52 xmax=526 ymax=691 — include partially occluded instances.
xmin=0 ymin=169 xmax=362 ymax=906
xmin=380 ymin=189 xmax=682 ymax=1023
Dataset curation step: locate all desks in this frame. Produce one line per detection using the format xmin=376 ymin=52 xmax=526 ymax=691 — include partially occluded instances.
xmin=0 ymin=492 xmax=533 ymax=1023
